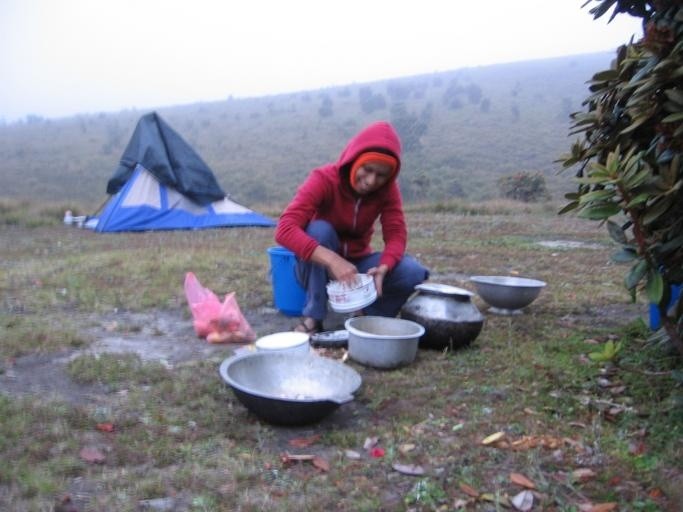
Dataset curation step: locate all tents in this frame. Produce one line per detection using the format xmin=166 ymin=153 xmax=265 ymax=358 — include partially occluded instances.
xmin=78 ymin=112 xmax=276 ymax=232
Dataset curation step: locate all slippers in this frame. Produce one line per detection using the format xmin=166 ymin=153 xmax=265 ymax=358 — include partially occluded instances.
xmin=291 ymin=321 xmax=322 ymax=334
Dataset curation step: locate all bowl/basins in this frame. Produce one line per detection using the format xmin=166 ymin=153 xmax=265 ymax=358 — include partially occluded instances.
xmin=469 ymin=274 xmax=548 ymax=316
xmin=344 ymin=316 xmax=425 ymax=369
xmin=327 ymin=273 xmax=376 ymax=312
xmin=254 ymin=331 xmax=310 ymax=354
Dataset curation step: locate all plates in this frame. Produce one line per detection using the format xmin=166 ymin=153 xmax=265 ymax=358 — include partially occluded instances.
xmin=311 ymin=329 xmax=348 ymax=348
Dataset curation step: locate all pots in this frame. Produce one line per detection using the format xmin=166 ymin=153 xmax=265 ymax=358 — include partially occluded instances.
xmin=218 ymin=351 xmax=361 ymax=426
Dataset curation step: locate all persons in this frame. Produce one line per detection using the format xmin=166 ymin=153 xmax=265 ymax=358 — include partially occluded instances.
xmin=275 ymin=122 xmax=430 ymax=333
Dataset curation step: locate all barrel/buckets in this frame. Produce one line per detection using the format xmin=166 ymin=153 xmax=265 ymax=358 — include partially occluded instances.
xmin=648 ymin=281 xmax=680 ymax=329
xmin=266 ymin=244 xmax=308 ymax=316
